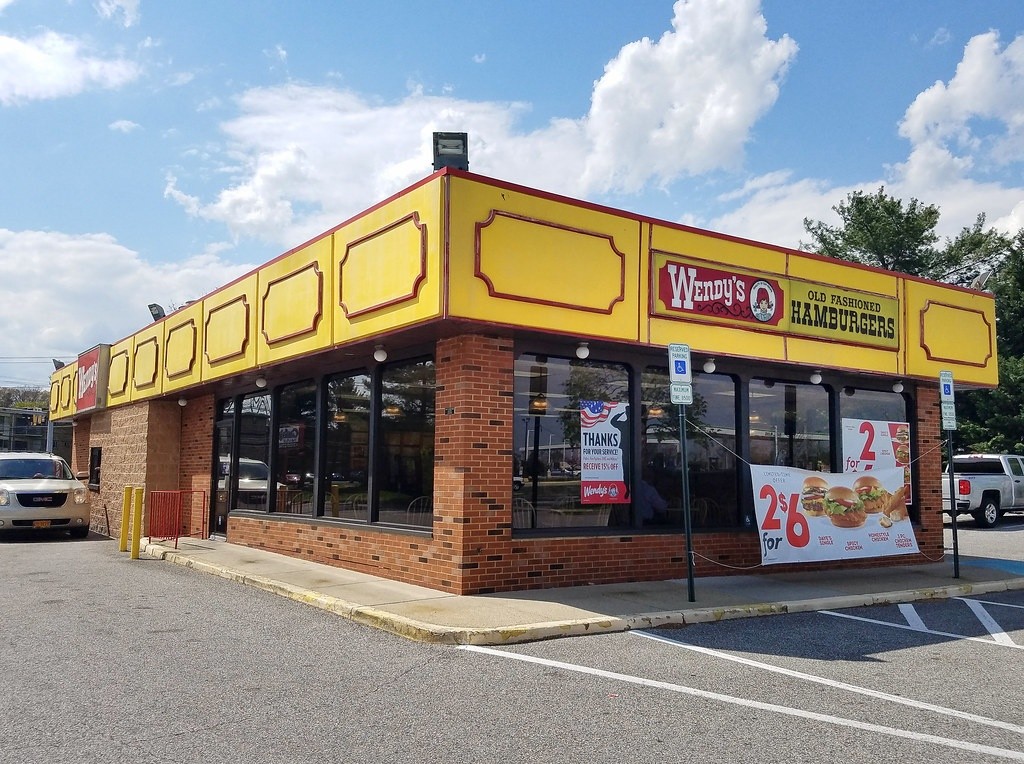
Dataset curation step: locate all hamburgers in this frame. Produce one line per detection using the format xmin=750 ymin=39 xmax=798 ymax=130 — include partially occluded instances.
xmin=895 ymin=425 xmax=910 ymax=483
xmin=800 ymin=476 xmax=884 ymax=527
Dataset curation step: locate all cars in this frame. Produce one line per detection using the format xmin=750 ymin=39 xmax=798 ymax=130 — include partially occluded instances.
xmin=0 ymin=452 xmax=91 ymax=540
xmin=216 ymin=456 xmax=288 ymax=510
xmin=285 ymin=472 xmax=313 ymax=484
xmin=349 ymin=470 xmax=366 ymax=483
xmin=329 ymin=472 xmax=344 ymax=480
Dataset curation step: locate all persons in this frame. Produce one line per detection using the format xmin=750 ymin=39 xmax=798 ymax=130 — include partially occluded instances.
xmin=639 ymin=469 xmax=683 ymax=529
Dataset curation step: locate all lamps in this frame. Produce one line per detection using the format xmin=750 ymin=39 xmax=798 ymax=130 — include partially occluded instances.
xmin=373 ymin=344 xmax=388 ymax=362
xmin=528 ymin=367 xmax=553 ymax=412
xmin=702 ymin=357 xmax=716 ymax=373
xmin=381 ymin=403 xmax=406 ymax=420
xmin=747 ymin=387 xmax=762 ymax=425
xmin=254 ymin=372 xmax=267 ymax=388
xmin=810 ymin=369 xmax=822 ymax=385
xmin=892 ymin=379 xmax=904 ymax=393
xmin=331 ymin=384 xmax=350 ymax=423
xmin=575 ymin=341 xmax=590 ymax=359
xmin=646 ymin=368 xmax=665 ymax=418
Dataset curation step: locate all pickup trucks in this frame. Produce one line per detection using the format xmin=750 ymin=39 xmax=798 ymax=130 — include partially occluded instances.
xmin=942 ymin=454 xmax=1024 ymax=528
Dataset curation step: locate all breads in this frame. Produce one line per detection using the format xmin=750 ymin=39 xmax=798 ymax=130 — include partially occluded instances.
xmin=880 ymin=487 xmax=906 ymax=528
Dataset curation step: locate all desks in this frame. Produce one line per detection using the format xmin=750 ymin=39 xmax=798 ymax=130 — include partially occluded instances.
xmin=663 ymin=507 xmax=700 ymax=529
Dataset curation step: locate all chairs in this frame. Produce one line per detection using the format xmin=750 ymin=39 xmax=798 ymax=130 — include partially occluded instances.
xmin=656 ymin=486 xmax=724 ymax=528
xmin=509 ymin=496 xmax=613 ymax=529
xmin=406 ymin=495 xmax=433 ymax=528
xmin=290 ymin=489 xmax=369 ymax=520
